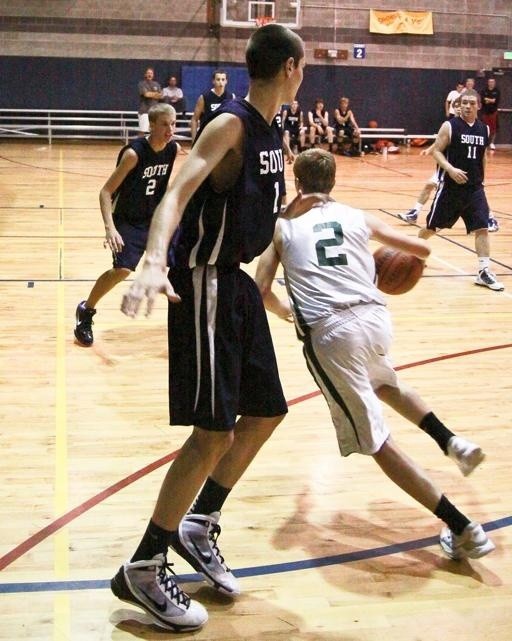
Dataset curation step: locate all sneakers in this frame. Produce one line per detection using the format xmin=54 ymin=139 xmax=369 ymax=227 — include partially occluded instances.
xmin=474 ymin=267 xmax=505 ymax=292
xmin=171 ymin=511 xmax=242 ymax=597
xmin=487 ymin=215 xmax=498 ymax=234
xmin=110 ymin=557 xmax=210 ymax=634
xmin=448 ymin=437 xmax=485 ymax=478
xmin=397 ymin=209 xmax=419 ymax=226
xmin=72 ymin=300 xmax=98 ymax=345
xmin=439 ymin=521 xmax=497 ymax=559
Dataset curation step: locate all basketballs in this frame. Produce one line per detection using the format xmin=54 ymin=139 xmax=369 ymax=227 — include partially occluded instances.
xmin=373 ymin=244 xmax=426 ymax=297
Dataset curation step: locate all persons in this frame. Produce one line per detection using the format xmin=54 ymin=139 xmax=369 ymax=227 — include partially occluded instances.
xmin=281 ymin=100 xmax=306 ymax=150
xmin=478 ymin=77 xmax=500 ymax=149
xmin=256 ymin=147 xmax=497 ymax=560
xmin=418 ymin=89 xmax=506 ymax=291
xmin=112 ymin=24 xmax=307 ymax=632
xmin=363 ymin=121 xmax=401 ymax=152
xmin=334 ymin=98 xmax=361 ymax=155
xmin=75 ymin=103 xmax=182 ymax=349
xmin=276 ymin=105 xmax=283 ymax=133
xmin=445 ymin=80 xmax=465 ymax=121
xmin=308 ymin=98 xmax=333 ymax=153
xmin=162 ymin=76 xmax=184 ymax=112
xmin=192 ymin=72 xmax=236 ymax=147
xmin=397 ymin=96 xmax=499 ymax=232
xmin=454 ymin=77 xmax=482 ymax=117
xmin=137 ymin=68 xmax=163 ymax=137
